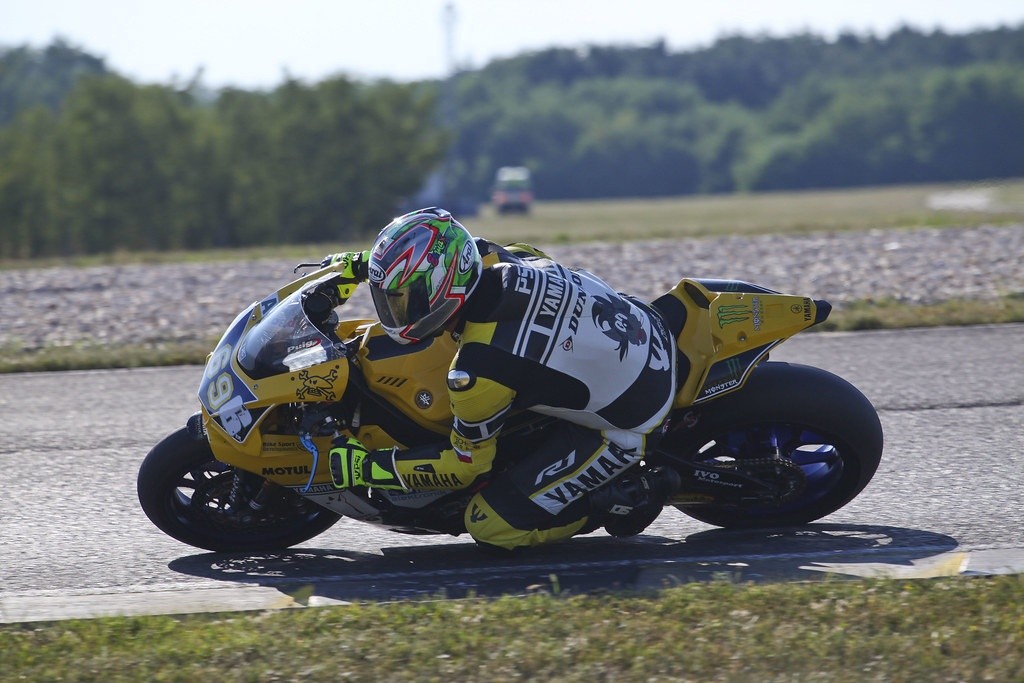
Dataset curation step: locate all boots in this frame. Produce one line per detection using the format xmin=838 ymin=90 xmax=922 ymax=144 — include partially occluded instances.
xmin=576 ymin=465 xmax=682 ymax=537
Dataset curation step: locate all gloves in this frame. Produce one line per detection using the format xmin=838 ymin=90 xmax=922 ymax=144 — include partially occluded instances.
xmin=328 ymin=437 xmax=412 ymax=493
xmin=320 ymin=250 xmax=370 ymax=305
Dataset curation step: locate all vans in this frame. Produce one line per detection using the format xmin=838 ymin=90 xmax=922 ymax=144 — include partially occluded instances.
xmin=493 ymin=166 xmax=531 ymax=214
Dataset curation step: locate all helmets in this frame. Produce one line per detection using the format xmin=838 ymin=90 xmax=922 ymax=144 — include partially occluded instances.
xmin=368 ymin=207 xmax=482 ymax=345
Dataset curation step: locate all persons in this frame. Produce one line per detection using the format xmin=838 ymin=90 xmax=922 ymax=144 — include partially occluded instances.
xmin=320 ymin=208 xmax=678 ymax=550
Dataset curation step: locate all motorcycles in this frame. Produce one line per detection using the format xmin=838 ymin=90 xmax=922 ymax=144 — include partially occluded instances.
xmin=136 ymin=257 xmax=884 ymax=560
